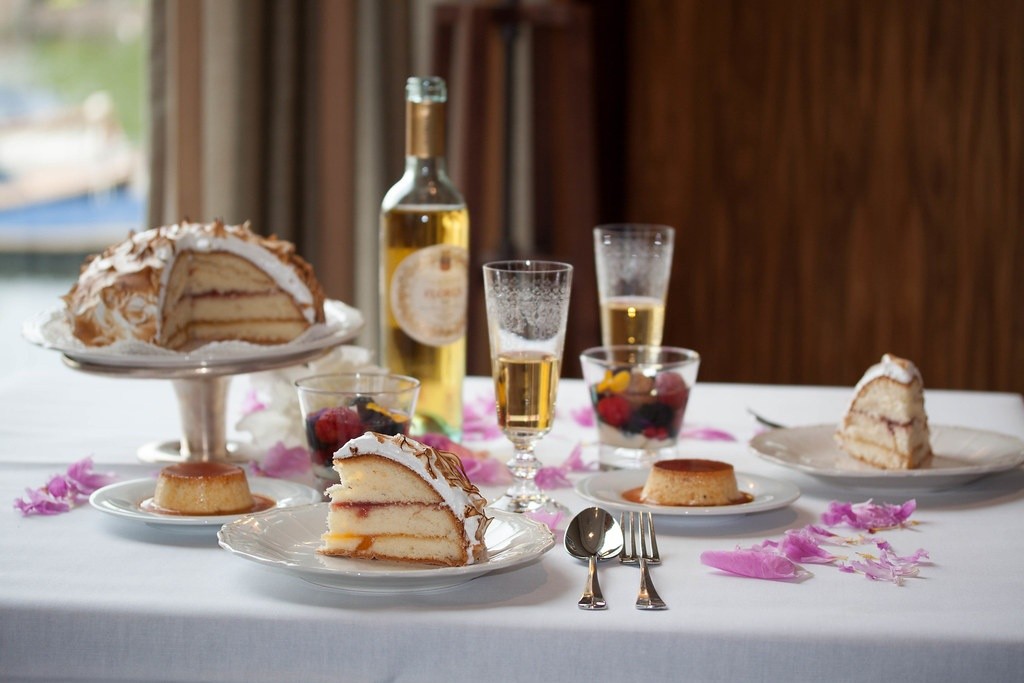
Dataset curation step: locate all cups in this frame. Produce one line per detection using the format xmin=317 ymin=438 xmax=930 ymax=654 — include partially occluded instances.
xmin=294 ymin=373 xmax=420 ymax=502
xmin=578 ymin=346 xmax=701 ymax=471
xmin=594 ymin=224 xmax=675 ymax=376
xmin=483 ymin=260 xmax=574 ymax=515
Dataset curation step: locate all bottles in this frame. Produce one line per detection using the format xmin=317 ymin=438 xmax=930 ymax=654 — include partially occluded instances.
xmin=378 ymin=77 xmax=471 ymax=443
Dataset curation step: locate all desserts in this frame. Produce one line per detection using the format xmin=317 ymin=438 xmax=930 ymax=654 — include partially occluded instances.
xmin=642 ymin=458 xmax=745 ymax=506
xmin=150 ymin=461 xmax=254 ymax=515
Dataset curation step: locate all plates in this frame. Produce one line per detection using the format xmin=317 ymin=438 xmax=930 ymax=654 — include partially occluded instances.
xmin=217 ymin=503 xmax=555 ymax=593
xmin=89 ymin=478 xmax=322 ymax=525
xmin=575 ymin=468 xmax=800 ymax=515
xmin=22 ymin=297 xmax=365 ymax=369
xmin=749 ymin=423 xmax=1023 ymax=487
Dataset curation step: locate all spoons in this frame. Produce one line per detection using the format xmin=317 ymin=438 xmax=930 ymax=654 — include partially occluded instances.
xmin=564 ymin=507 xmax=624 ymax=609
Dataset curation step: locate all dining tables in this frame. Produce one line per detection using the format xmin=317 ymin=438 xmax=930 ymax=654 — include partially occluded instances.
xmin=0 ymin=377 xmax=1024 ymax=683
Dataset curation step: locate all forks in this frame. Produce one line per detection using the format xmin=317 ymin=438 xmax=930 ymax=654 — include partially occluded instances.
xmin=619 ymin=511 xmax=667 ymax=609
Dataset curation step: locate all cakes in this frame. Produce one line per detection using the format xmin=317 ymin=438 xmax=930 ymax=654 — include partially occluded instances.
xmin=834 ymin=352 xmax=932 ymax=472
xmin=59 ymin=218 xmax=326 ymax=348
xmin=321 ymin=431 xmax=496 ymax=567
